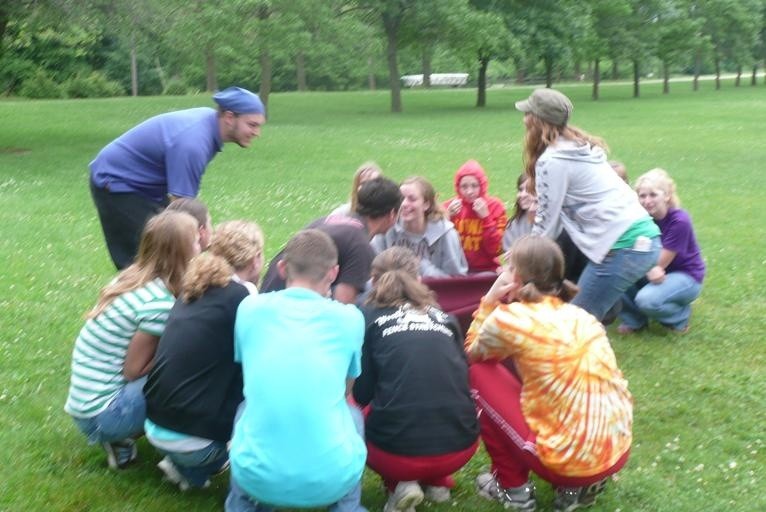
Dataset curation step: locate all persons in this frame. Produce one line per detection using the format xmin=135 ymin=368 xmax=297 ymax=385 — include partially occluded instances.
xmin=463 ymin=238 xmax=635 ymax=512
xmin=225 ymin=230 xmax=367 ymax=511
xmin=85 ymin=83 xmax=264 ymax=286
xmin=515 ymin=83 xmax=662 ymax=321
xmin=351 ymin=244 xmax=481 ymax=510
xmin=260 ymin=156 xmax=706 ymax=334
xmin=63 ymin=213 xmax=203 ymax=471
xmin=143 ymin=220 xmax=263 ymax=491
xmin=161 ymin=198 xmax=211 ymax=252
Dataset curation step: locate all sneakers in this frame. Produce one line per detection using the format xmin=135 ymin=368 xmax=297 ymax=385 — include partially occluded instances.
xmin=330 ymin=504 xmax=369 ymax=512
xmin=425 ymin=483 xmax=451 ymax=503
xmin=103 ymin=438 xmax=138 ymax=468
xmin=552 ymin=476 xmax=607 ymax=512
xmin=383 ymin=480 xmax=425 ymax=512
xmin=158 ymin=456 xmax=212 ymax=492
xmin=616 ymin=322 xmax=644 ymax=335
xmin=224 ymin=490 xmax=257 ymax=512
xmin=475 ymin=473 xmax=538 ymax=512
xmin=661 ymin=323 xmax=691 ymax=336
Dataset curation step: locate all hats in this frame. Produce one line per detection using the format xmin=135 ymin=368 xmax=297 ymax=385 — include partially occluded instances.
xmin=515 ymin=88 xmax=573 ymax=126
xmin=211 ymin=86 xmax=265 ymax=114
